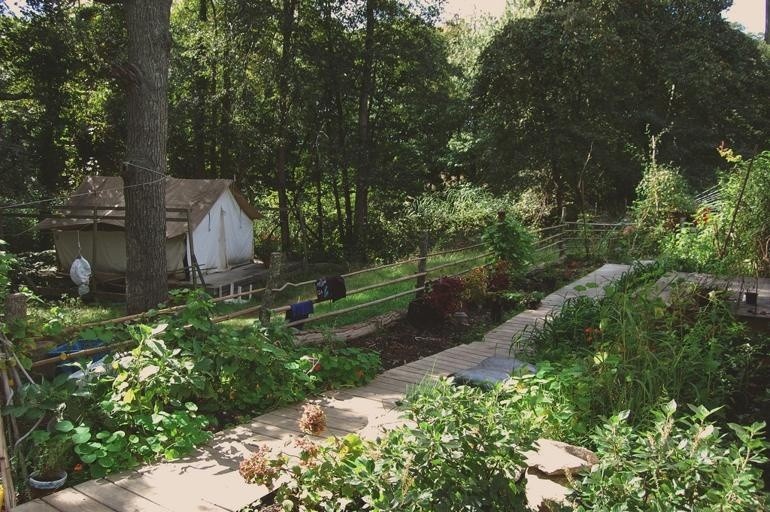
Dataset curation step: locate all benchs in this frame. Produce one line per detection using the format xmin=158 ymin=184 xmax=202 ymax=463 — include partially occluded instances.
xmin=457 ymin=354 xmax=535 ymax=391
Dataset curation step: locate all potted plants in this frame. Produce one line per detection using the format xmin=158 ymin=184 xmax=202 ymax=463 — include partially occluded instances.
xmin=29 ymin=444 xmax=67 ymax=500
